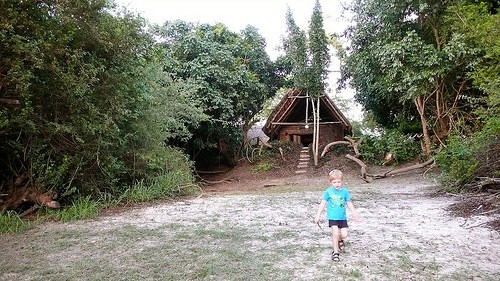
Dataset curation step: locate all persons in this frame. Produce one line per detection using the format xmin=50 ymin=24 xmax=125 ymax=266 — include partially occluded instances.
xmin=314 ymin=170 xmax=357 ymax=262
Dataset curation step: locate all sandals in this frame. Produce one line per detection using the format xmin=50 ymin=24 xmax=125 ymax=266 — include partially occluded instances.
xmin=338 ymin=240 xmax=345 ymax=251
xmin=332 ymin=251 xmax=340 ymax=262
